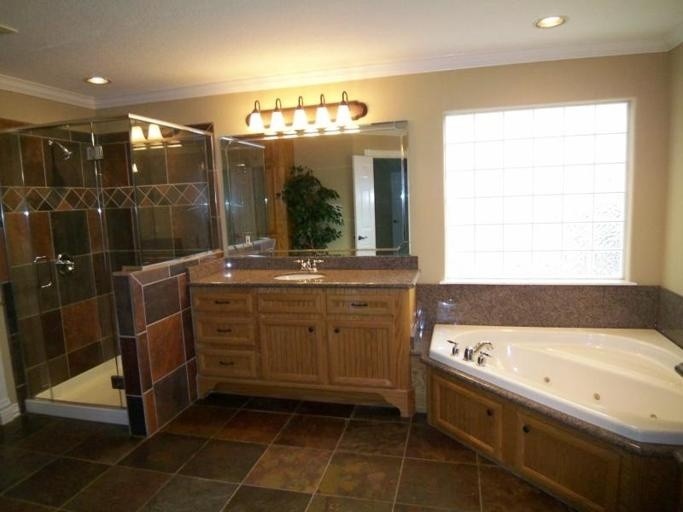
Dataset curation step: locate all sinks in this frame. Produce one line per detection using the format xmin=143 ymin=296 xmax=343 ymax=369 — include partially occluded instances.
xmin=273 ymin=272 xmax=325 ymax=280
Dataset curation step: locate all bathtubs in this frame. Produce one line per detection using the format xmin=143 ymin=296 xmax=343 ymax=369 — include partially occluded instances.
xmin=429 ymin=325 xmax=683 ymax=444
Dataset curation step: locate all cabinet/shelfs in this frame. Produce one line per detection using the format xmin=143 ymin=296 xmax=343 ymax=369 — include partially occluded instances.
xmin=189 ymin=287 xmax=417 ymax=418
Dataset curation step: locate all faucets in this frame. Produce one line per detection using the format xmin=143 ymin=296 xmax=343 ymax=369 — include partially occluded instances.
xmin=464 ymin=340 xmax=494 ymax=357
xmin=301 ymin=260 xmax=311 ymax=270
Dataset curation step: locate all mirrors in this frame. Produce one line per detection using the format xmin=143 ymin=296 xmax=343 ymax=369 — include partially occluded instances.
xmin=220 ymin=120 xmax=409 ymax=257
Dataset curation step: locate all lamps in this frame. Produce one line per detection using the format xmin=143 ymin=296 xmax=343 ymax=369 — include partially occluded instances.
xmin=244 ymin=91 xmax=367 ymax=135
xmin=130 ymin=121 xmax=192 ymax=150
